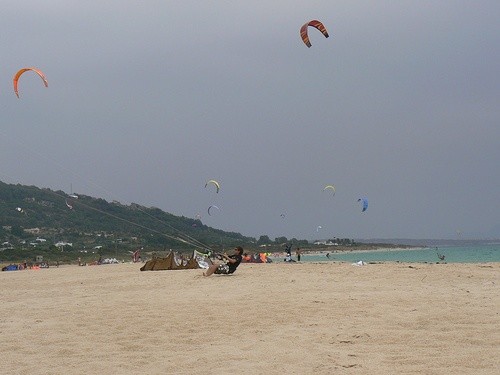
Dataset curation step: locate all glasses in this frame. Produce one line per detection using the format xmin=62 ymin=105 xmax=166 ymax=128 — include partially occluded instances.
xmin=235 ymin=249 xmax=237 ymax=251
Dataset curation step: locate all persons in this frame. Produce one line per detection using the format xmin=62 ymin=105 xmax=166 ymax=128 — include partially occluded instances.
xmin=296 ymin=247 xmax=302 ymax=261
xmin=198 ymin=246 xmax=243 ymax=276
xmin=284 ymin=243 xmax=293 ymax=257
